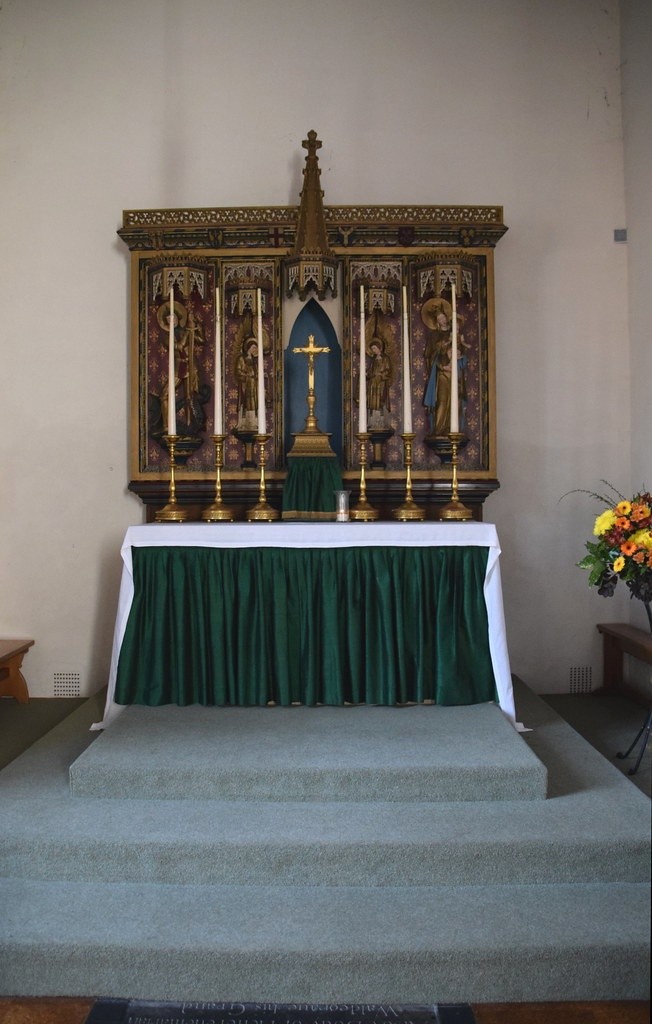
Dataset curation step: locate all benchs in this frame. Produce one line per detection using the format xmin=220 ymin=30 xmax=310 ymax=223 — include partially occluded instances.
xmin=0 ymin=640 xmax=34 ymax=704
xmin=591 ymin=623 xmax=652 ymax=709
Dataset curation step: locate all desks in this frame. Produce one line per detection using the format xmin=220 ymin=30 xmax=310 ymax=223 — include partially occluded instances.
xmin=90 ymin=520 xmax=533 ymax=731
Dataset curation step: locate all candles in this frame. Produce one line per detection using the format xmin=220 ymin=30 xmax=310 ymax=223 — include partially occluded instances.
xmin=359 ymin=286 xmax=367 ymax=433
xmin=168 ymin=289 xmax=176 ymax=435
xmin=257 ymin=288 xmax=266 ymax=434
xmin=215 ymin=287 xmax=222 ymax=435
xmin=403 ymin=286 xmax=412 ymax=432
xmin=450 ymin=284 xmax=458 ymax=432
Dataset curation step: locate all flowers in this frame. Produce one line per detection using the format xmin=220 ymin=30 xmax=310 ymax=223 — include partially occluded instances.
xmin=558 ymin=480 xmax=652 ymax=605
